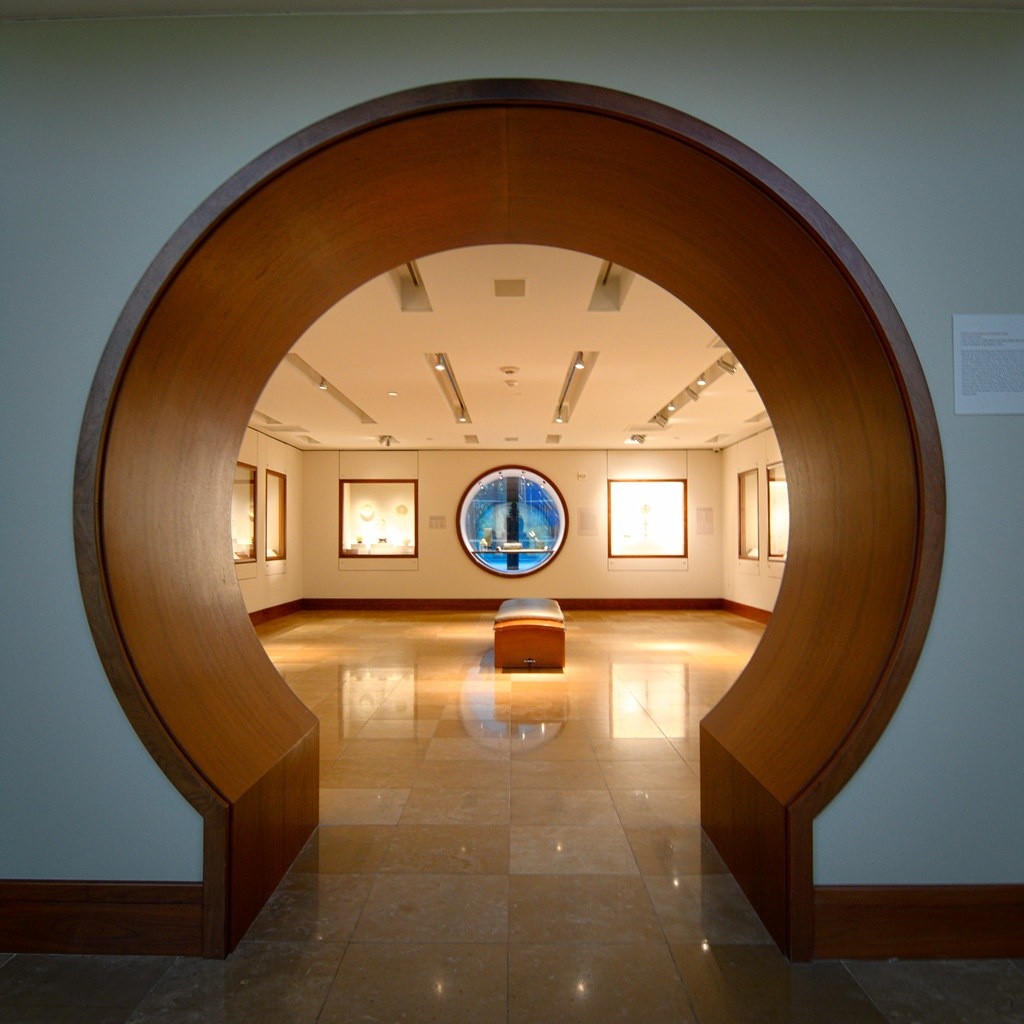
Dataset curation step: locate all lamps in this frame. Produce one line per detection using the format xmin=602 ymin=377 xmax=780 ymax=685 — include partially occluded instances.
xmin=556 ymin=413 xmax=564 ymax=425
xmin=697 ymin=373 xmax=707 ymax=386
xmin=684 ymin=387 xmax=700 ymax=403
xmin=318 ymin=377 xmax=327 ymax=390
xmin=434 ymin=353 xmax=445 ymax=371
xmin=459 ymin=410 xmax=466 ymax=421
xmin=667 ymin=400 xmax=674 ymax=411
xmin=574 ymin=353 xmax=586 ymax=370
xmin=653 ymin=412 xmax=669 ymax=429
xmin=379 ymin=436 xmax=391 ymax=447
xmin=630 ymin=434 xmax=646 ymax=447
xmin=716 ymin=359 xmax=737 ymax=377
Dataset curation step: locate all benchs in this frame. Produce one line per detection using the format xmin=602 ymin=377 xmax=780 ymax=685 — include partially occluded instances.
xmin=492 ymin=597 xmax=566 ymax=670
